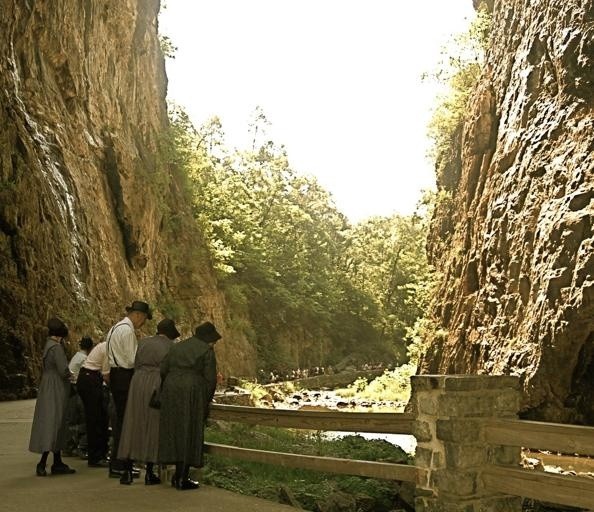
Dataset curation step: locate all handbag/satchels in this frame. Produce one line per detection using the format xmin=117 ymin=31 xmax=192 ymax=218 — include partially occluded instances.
xmin=148 ymin=390 xmax=161 ymax=410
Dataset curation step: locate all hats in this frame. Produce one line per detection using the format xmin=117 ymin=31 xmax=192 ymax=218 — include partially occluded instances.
xmin=47 ymin=318 xmax=69 ymax=338
xmin=79 ymin=336 xmax=97 ymax=347
xmin=126 ymin=300 xmax=152 ymax=321
xmin=195 ymin=322 xmax=222 ymax=344
xmin=156 ymin=318 xmax=180 ymax=340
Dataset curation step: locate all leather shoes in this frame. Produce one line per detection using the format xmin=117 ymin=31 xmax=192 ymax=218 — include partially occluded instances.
xmin=171 ymin=472 xmax=200 ymax=491
xmin=145 ymin=472 xmax=162 ymax=485
xmin=36 ymin=463 xmax=47 ymax=477
xmin=62 ymin=448 xmax=141 ymax=485
xmin=51 ymin=463 xmax=75 ymax=475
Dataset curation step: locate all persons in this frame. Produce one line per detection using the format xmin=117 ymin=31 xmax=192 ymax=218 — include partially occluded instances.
xmin=76 ymin=341 xmax=110 ymax=467
xmin=361 ymin=361 xmax=370 ymax=371
xmin=259 ymin=365 xmax=333 ymax=385
xmin=105 ymin=300 xmax=153 ymax=479
xmin=216 ymin=372 xmax=223 ymax=392
xmin=68 ymin=335 xmax=94 ymax=458
xmin=27 ymin=315 xmax=78 ymax=477
xmin=159 ymin=320 xmax=223 ymax=491
xmin=116 ymin=318 xmax=181 ymax=485
